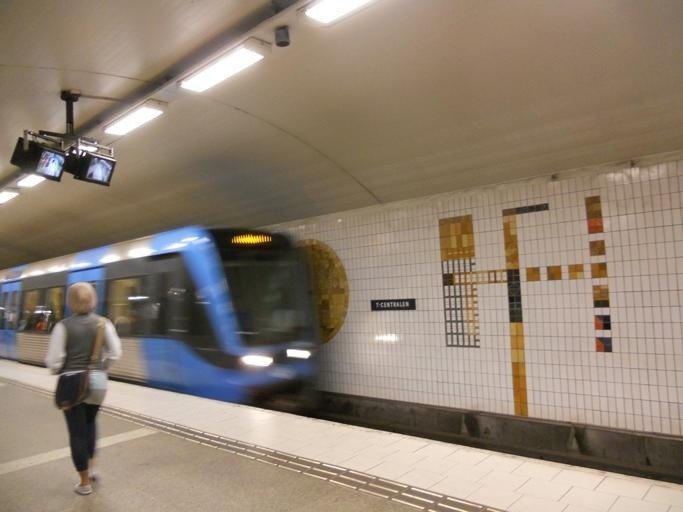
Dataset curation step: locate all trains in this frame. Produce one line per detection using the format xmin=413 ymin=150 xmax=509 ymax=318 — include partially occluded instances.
xmin=0 ymin=224 xmax=322 ymax=411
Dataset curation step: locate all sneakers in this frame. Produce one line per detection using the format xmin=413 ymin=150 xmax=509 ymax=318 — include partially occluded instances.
xmin=75 ymin=470 xmax=101 ymax=495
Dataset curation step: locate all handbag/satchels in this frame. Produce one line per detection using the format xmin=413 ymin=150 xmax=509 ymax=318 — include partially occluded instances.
xmin=55 ymin=373 xmax=92 ymax=410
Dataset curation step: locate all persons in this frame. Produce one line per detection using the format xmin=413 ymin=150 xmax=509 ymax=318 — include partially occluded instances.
xmin=43 ymin=281 xmax=124 ymax=497
xmin=40 ymin=153 xmax=57 ymax=175
xmin=115 ymin=304 xmax=131 ymax=337
xmin=36 ymin=312 xmax=48 ymax=331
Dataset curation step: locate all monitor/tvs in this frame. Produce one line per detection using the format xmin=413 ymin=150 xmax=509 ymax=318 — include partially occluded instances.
xmin=9 ymin=136 xmax=118 ymax=187
